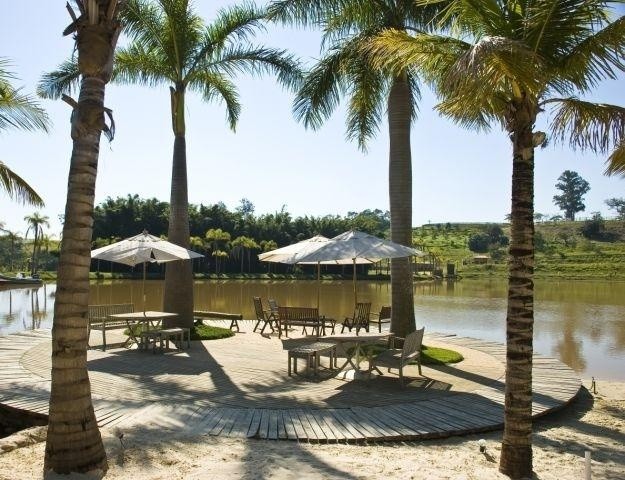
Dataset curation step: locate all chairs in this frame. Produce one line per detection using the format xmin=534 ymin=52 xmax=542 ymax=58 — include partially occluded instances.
xmin=340 ymin=302 xmax=371 ymax=333
xmin=369 ymin=306 xmax=392 ymax=332
xmin=367 ymin=327 xmax=424 ymax=383
xmin=268 ymin=299 xmax=291 ymax=329
xmin=252 ymin=298 xmax=282 ymax=334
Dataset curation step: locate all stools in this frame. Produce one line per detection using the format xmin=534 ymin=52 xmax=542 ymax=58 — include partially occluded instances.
xmin=279 ymin=336 xmax=338 ymax=379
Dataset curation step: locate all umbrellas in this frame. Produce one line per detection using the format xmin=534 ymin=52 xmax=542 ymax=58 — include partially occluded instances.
xmin=284 ymin=226 xmax=424 ymax=336
xmin=89 ymin=230 xmax=205 ymax=314
xmin=258 ymin=235 xmax=382 ymax=318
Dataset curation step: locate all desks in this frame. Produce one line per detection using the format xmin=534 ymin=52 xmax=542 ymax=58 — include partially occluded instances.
xmin=109 ymin=311 xmax=179 ymax=351
xmin=316 ymin=330 xmax=396 ymax=383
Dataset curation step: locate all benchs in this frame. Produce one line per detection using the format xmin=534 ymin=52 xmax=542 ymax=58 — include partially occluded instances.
xmin=87 ymin=303 xmax=135 ymax=352
xmin=193 ymin=310 xmax=243 ymax=332
xmin=301 ymin=317 xmax=336 ymax=336
xmin=138 ymin=328 xmax=191 ymax=353
xmin=277 ymin=306 xmax=322 ymax=340
